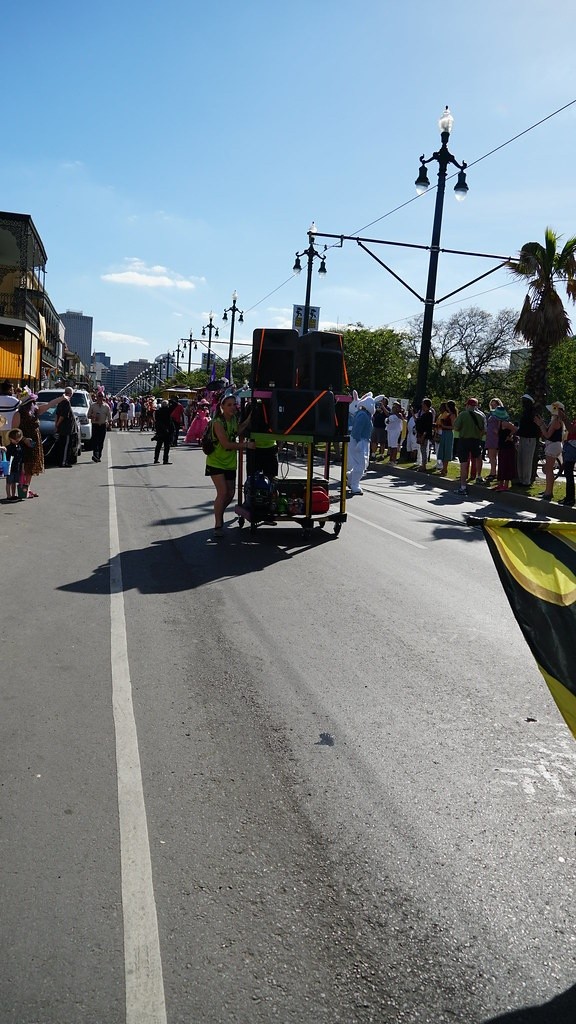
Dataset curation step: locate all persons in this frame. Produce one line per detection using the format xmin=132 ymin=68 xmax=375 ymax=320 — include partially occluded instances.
xmin=205 ymin=394 xmax=256 ymax=537
xmin=87 ymin=391 xmax=113 ymax=463
xmin=345 ymin=395 xmax=576 ymax=506
xmin=104 ymin=395 xmax=212 ymax=445
xmin=0 ymin=382 xmax=75 ymax=502
xmin=347 ymin=389 xmax=385 ymax=495
xmin=154 ymin=400 xmax=177 ymax=464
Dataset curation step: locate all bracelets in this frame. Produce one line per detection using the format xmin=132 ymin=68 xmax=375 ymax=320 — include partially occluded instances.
xmin=246 ymin=442 xmax=248 ymax=448
xmin=109 ymin=424 xmax=112 ymax=426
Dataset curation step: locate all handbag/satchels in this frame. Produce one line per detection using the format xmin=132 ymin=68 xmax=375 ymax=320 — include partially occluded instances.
xmin=434 ymin=433 xmax=442 ymax=443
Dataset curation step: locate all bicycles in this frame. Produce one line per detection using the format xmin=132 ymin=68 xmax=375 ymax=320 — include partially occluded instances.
xmin=535 ymin=440 xmax=562 ymax=481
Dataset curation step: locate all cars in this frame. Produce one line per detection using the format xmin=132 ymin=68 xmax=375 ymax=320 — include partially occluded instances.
xmin=32 ymin=402 xmax=81 ymax=464
xmin=537 ymin=442 xmax=576 ymax=470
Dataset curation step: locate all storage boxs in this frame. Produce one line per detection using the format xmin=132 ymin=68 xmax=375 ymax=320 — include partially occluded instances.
xmin=273 ymin=475 xmax=329 ymax=498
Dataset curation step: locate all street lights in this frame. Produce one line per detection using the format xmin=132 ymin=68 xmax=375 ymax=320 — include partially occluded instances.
xmin=201 ymin=309 xmax=219 ymax=374
xmin=400 ymin=105 xmax=469 ymax=461
xmin=222 ymin=289 xmax=243 ymax=386
xmin=441 ymin=369 xmax=446 ymax=394
xmin=293 ymin=220 xmax=327 ymax=335
xmin=183 ymin=327 xmax=198 ymax=375
xmin=461 ymin=369 xmax=466 ymax=386
xmin=126 ymin=340 xmax=184 ymax=398
xmin=406 ymin=372 xmax=411 ymax=398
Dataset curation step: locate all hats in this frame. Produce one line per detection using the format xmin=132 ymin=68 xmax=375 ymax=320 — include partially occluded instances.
xmin=463 ymin=399 xmax=478 ymax=406
xmin=491 ymin=406 xmax=509 ymax=421
xmin=520 ymin=394 xmax=537 ymax=404
xmin=199 ymin=399 xmax=209 ymax=405
xmin=546 ymin=401 xmax=564 ymax=415
xmin=6 ymin=428 xmax=23 ymax=442
xmin=16 ymin=385 xmax=38 ymax=408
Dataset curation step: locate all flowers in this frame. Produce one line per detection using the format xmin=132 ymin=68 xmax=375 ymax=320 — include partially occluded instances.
xmin=18 ymin=390 xmax=30 ymax=400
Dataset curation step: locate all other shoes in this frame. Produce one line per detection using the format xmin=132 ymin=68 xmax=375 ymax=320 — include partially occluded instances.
xmin=345 ymin=471 xmax=352 ymax=490
xmin=371 ymin=455 xmax=575 ymax=507
xmin=154 ymin=459 xmax=160 ymax=464
xmin=163 ymin=460 xmax=172 ymax=465
xmin=6 ymin=495 xmax=21 ymax=502
xmin=92 ymin=455 xmax=101 ymax=463
xmin=27 ymin=491 xmax=39 ymax=498
xmin=265 ymin=521 xmax=277 ymax=525
xmin=351 ymin=489 xmax=363 ymax=495
xmin=213 ymin=523 xmax=226 ymax=538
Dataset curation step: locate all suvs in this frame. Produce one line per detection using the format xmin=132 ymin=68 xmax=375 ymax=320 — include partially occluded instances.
xmin=34 ymin=387 xmax=93 ymax=448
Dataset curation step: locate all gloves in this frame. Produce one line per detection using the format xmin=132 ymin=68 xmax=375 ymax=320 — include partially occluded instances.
xmin=356 ymin=440 xmax=363 ymax=450
xmin=505 ymin=435 xmax=514 ymax=443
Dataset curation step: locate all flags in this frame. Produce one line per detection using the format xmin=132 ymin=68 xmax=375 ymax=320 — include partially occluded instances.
xmin=55 ymin=368 xmax=58 ymax=375
xmin=46 ymin=368 xmax=50 ymax=377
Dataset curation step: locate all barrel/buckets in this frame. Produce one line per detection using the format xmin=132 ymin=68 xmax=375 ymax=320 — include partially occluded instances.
xmin=0 ymin=461 xmax=11 ymax=475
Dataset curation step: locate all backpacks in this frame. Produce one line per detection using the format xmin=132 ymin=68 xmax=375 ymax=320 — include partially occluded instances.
xmin=201 ymin=415 xmax=239 ymax=455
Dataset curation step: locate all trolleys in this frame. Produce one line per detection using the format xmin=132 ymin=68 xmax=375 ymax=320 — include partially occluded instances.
xmin=234 ymin=434 xmax=350 ymax=543
